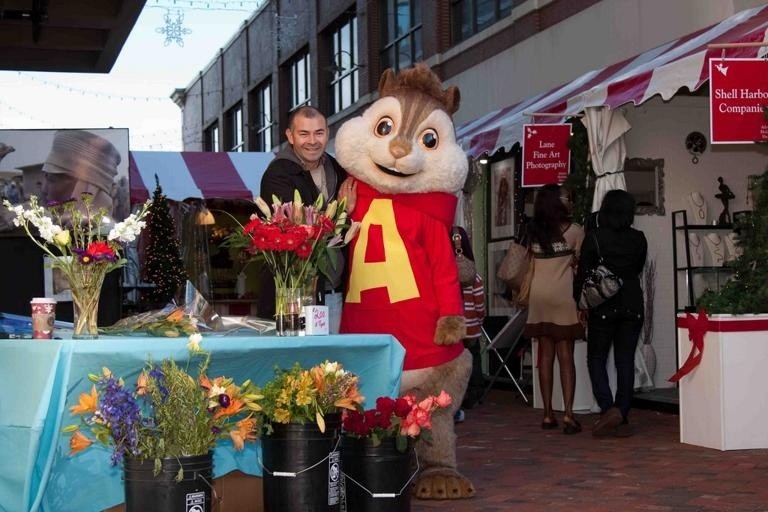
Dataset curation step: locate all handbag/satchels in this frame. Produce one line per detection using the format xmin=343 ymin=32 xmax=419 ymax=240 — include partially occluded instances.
xmin=510 ymin=255 xmax=535 ymax=309
xmin=452 ymin=227 xmax=477 ymax=284
xmin=578 ymin=229 xmax=623 ymax=310
xmin=496 ymin=220 xmax=533 ymax=287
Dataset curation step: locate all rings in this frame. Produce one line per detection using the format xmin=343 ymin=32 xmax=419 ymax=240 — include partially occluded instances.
xmin=348 ymin=185 xmax=353 ymax=192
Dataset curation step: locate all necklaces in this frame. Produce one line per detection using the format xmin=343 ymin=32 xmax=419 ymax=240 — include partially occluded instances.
xmin=690 ymin=192 xmax=705 ymax=220
xmin=689 ymin=235 xmax=702 ymax=260
xmin=728 ymin=235 xmax=742 ymax=254
xmin=705 ymin=234 xmax=724 ymax=263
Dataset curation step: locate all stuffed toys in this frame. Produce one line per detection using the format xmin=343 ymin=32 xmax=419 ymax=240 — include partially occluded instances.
xmin=334 ymin=62 xmax=475 ymax=500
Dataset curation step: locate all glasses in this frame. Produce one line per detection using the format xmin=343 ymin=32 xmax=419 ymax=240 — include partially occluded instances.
xmin=560 ymin=195 xmax=571 ymax=201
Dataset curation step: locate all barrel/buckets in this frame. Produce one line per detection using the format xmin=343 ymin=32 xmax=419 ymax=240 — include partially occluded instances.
xmin=121 ymin=453 xmax=223 ymax=511
xmin=340 ymin=437 xmax=419 ymax=512
xmin=256 ymin=413 xmax=342 ymax=512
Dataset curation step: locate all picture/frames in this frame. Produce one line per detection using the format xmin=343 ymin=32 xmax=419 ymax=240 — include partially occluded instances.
xmin=488 ymin=240 xmax=514 ymax=316
xmin=487 ymin=155 xmax=516 ymax=239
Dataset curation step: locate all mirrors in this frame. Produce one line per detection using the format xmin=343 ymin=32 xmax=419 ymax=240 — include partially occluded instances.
xmin=625 ymin=157 xmax=665 ymax=216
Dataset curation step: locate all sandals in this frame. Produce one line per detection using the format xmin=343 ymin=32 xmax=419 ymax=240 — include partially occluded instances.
xmin=563 ymin=417 xmax=582 ymax=432
xmin=543 ymin=417 xmax=557 ymax=428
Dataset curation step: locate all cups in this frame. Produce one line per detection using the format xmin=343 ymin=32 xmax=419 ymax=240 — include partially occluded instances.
xmin=30 ymin=298 xmax=55 ymax=338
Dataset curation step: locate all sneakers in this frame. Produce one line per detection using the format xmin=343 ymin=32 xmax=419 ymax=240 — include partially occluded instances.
xmin=592 ymin=407 xmax=622 ymax=435
xmin=617 ymin=424 xmax=631 ymax=436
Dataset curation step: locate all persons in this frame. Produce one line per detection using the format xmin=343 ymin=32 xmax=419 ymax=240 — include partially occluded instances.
xmin=446 ymin=228 xmax=485 ymax=424
xmin=0 ymin=170 xmax=47 ymax=202
xmin=507 ymin=183 xmax=587 ymax=435
xmin=573 ymin=190 xmax=648 ymax=439
xmin=259 ymin=105 xmax=359 ymax=319
xmin=713 ymin=176 xmax=737 ymax=226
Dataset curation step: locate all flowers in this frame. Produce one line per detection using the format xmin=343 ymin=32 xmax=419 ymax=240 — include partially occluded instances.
xmin=246 ymin=360 xmax=365 ymax=433
xmin=241 ymin=189 xmax=362 ymax=337
xmin=98 ymin=305 xmax=199 ymax=338
xmin=62 ymin=333 xmax=264 ymax=482
xmin=342 ymin=389 xmax=452 ymax=453
xmin=2 ymin=193 xmax=152 ymax=334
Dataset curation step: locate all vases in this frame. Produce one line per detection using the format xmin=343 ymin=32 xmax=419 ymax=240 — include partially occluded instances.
xmin=68 ymin=273 xmax=105 ymax=340
xmin=292 ymin=276 xmax=317 ymax=336
xmin=276 ymin=288 xmax=300 ymax=336
xmin=640 ymin=345 xmax=656 ymax=393
